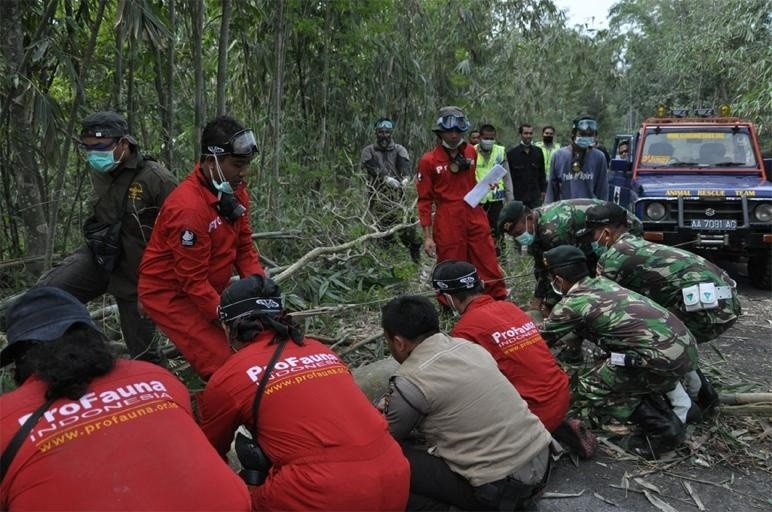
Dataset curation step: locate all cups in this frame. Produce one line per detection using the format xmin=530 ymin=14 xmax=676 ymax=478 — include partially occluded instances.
xmin=438 ymin=116 xmax=468 ymax=132
xmin=229 ymin=128 xmax=260 ymax=158
xmin=83 ymin=143 xmax=119 ymax=150
xmin=573 ymin=120 xmax=597 ymax=131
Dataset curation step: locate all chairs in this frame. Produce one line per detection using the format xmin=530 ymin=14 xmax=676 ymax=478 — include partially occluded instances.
xmin=562 ymin=420 xmax=596 ymax=459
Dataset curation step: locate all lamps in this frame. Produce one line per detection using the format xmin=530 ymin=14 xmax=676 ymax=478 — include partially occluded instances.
xmin=687 ymin=142 xmax=733 ymax=166
xmin=644 ymin=141 xmax=680 ymax=165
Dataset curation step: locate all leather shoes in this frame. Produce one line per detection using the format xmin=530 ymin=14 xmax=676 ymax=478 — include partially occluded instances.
xmin=384 ymin=175 xmax=400 ymax=190
xmin=402 ymin=179 xmax=409 ymax=190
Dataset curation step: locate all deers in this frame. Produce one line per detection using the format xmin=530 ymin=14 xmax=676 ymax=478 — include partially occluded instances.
xmin=515 ymin=231 xmax=535 ymax=246
xmin=590 ymin=241 xmax=607 ymax=257
xmin=574 ymin=136 xmax=592 ymax=148
xmin=543 ymin=136 xmax=553 ymax=143
xmin=482 ymin=140 xmax=496 ymax=150
xmin=550 ymin=281 xmax=563 ymax=296
xmin=212 ymin=182 xmax=237 ymax=193
xmin=88 ymin=151 xmax=122 ymax=173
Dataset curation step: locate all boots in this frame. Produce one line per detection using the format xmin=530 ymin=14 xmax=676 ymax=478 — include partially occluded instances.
xmin=608 ymin=105 xmax=772 ymax=285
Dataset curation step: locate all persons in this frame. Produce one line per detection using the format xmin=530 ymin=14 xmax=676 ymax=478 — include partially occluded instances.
xmin=1 ymin=288 xmax=252 ymax=511
xmin=199 ymin=275 xmax=411 ymax=511
xmin=499 ymin=199 xmax=642 ymax=366
xmin=378 ymin=293 xmax=560 ymax=512
xmin=433 ymin=259 xmax=596 ymax=457
xmin=619 ymin=141 xmax=629 ymax=158
xmin=44 ymin=110 xmax=178 ymax=371
xmin=360 ymin=118 xmax=422 ymax=264
xmin=468 ymin=125 xmax=514 ymax=255
xmin=507 ymin=115 xmax=611 ymax=254
xmin=587 ymin=203 xmax=739 ymax=414
xmin=415 ymin=107 xmax=509 ymax=312
xmin=538 ymin=245 xmax=721 ymax=457
xmin=139 ymin=116 xmax=266 ymax=379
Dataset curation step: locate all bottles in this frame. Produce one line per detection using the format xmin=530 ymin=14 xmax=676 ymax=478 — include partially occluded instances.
xmin=497 ymin=201 xmax=523 ymax=232
xmin=1 ymin=285 xmax=106 ymax=367
xmin=575 ymin=203 xmax=626 ymax=238
xmin=543 ymin=245 xmax=587 ymax=269
xmin=82 ymin=112 xmax=138 ymax=146
xmin=432 ymin=106 xmax=470 ymax=133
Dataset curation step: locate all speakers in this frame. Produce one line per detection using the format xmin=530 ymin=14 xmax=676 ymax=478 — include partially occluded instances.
xmin=624 ymin=366 xmax=719 ymax=458
